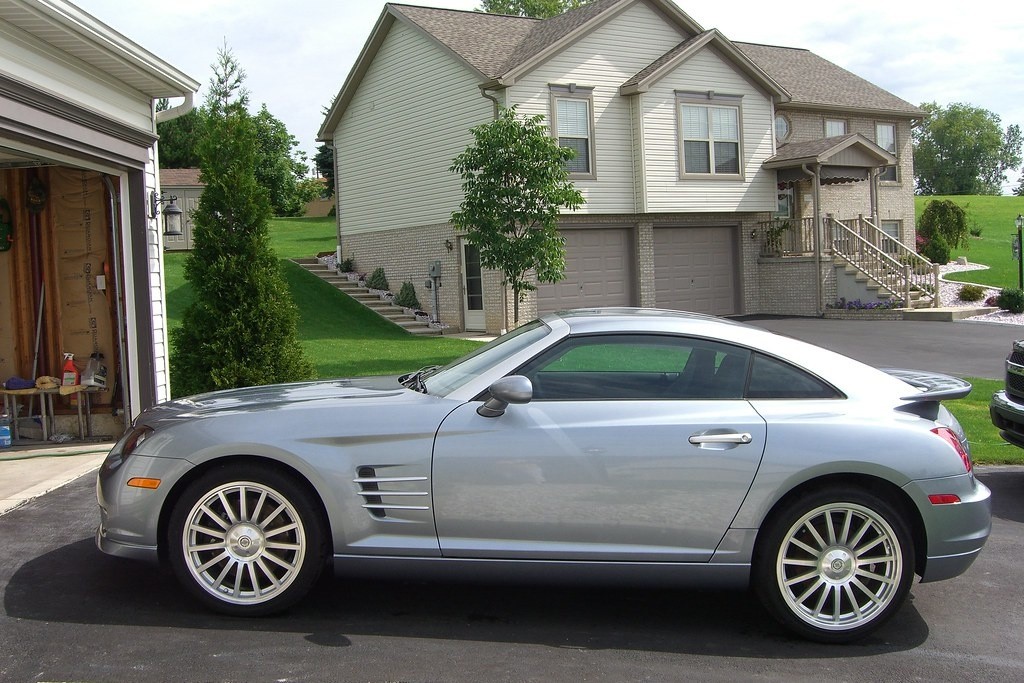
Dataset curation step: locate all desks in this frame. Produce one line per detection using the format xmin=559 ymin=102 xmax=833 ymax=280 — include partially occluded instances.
xmin=0 ymin=385 xmax=113 ymax=443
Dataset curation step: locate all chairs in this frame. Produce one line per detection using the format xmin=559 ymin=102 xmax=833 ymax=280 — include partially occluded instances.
xmin=661 ymin=346 xmax=754 ymax=399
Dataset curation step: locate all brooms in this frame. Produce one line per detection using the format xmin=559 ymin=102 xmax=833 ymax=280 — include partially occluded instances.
xmin=19 ymin=280 xmax=44 ymax=439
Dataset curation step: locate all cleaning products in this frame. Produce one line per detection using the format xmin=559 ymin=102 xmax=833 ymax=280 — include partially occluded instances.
xmin=62 ymin=352 xmax=78 ymax=386
xmin=0 ymin=415 xmax=12 ymax=448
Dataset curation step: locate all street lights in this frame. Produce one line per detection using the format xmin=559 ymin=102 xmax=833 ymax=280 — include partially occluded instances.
xmin=1015 ymin=213 xmax=1024 ymax=291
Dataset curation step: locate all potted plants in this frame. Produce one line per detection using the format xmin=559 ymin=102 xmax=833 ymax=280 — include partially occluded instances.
xmin=762 ymin=219 xmax=795 ymax=253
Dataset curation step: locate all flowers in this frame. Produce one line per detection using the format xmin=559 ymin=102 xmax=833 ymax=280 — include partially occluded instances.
xmin=825 ymin=294 xmax=907 ymax=309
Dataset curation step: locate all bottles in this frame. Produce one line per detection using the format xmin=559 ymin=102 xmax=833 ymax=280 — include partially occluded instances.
xmin=0 ymin=414 xmax=12 ymax=448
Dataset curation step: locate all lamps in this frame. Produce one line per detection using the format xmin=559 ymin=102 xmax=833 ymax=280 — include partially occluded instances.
xmin=444 ymin=240 xmax=453 ymax=254
xmin=751 ymin=229 xmax=760 ymax=239
xmin=1013 ymin=213 xmax=1024 ymax=228
xmin=149 ymin=187 xmax=184 ymax=235
xmin=1010 ymin=234 xmax=1020 ymax=261
xmin=26 ymin=176 xmax=48 ymax=213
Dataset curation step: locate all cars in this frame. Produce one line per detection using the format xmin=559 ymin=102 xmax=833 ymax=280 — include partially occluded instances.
xmin=94 ymin=306 xmax=992 ymax=640
xmin=990 ymin=339 xmax=1024 ymax=449
